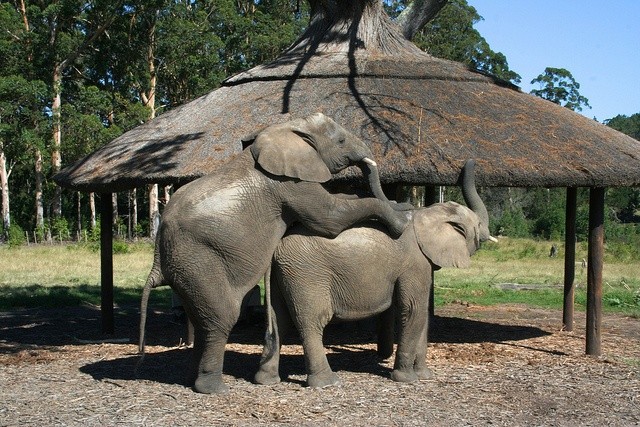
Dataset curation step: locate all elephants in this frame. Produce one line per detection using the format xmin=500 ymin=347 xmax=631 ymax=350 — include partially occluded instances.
xmin=253 ymin=158 xmax=500 ymax=388
xmin=138 ymin=112 xmax=416 ymax=395
